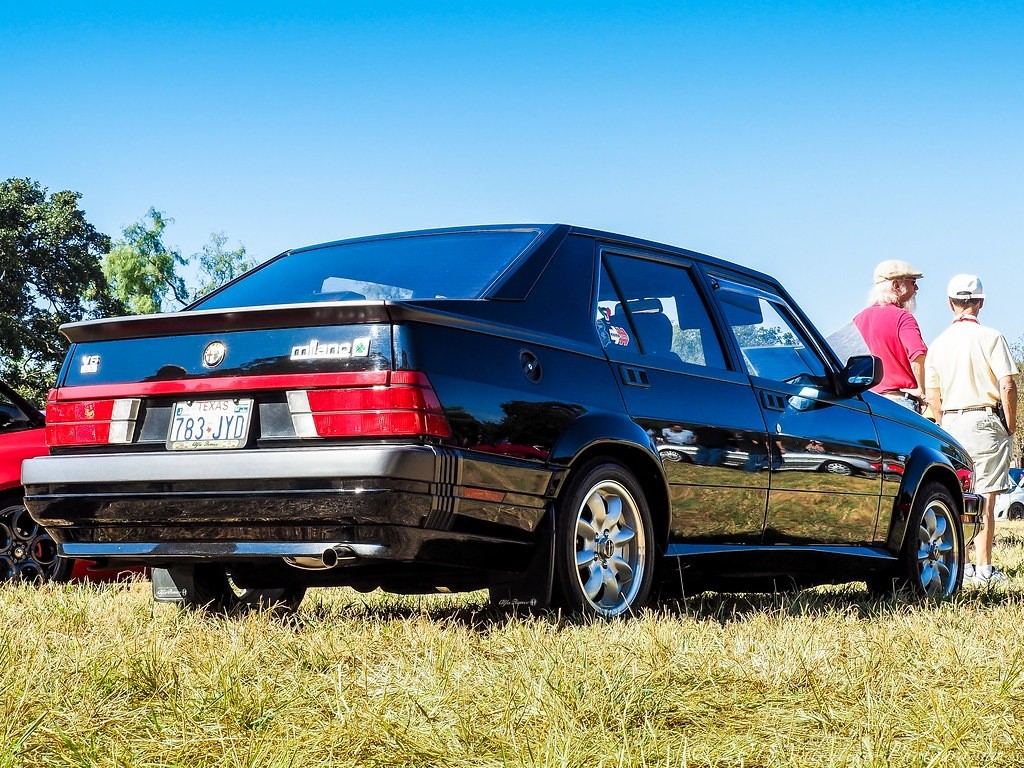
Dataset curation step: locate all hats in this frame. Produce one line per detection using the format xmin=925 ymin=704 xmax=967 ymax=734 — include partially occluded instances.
xmin=875 ymin=260 xmax=923 ymax=282
xmin=947 ymin=273 xmax=985 ymax=299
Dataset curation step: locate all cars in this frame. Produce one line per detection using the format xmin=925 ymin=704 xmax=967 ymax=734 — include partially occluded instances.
xmin=984 ymin=476 xmax=1024 ymax=523
xmin=18 ymin=224 xmax=987 ymax=628
xmin=1 ymin=380 xmax=152 ymax=589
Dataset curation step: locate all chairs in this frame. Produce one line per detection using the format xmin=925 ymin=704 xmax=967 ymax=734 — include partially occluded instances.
xmin=598 ymin=312 xmax=684 ymax=364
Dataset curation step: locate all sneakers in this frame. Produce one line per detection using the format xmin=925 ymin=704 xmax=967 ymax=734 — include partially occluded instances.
xmin=974 ymin=566 xmax=1009 ymax=586
xmin=964 ymin=562 xmax=975 ymax=580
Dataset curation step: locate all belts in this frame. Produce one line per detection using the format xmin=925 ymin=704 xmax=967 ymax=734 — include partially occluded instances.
xmin=881 ymin=390 xmax=928 ymax=406
xmin=942 ymin=407 xmax=997 ymax=414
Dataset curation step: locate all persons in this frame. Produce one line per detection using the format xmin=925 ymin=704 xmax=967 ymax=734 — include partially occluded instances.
xmin=852 ymin=260 xmax=928 ymax=415
xmin=923 ymin=274 xmax=1019 ymax=585
